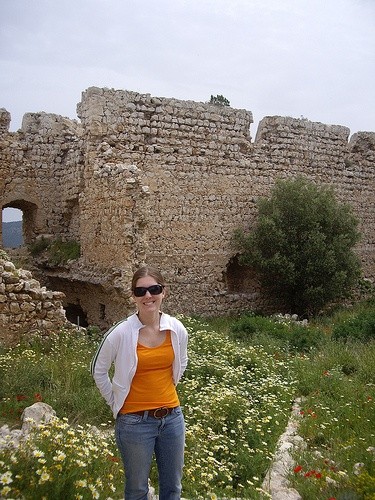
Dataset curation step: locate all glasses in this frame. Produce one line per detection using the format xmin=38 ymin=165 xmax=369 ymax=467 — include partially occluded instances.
xmin=131 ymin=284 xmax=164 ymax=297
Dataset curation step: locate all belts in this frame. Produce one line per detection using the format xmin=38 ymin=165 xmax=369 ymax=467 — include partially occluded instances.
xmin=130 ymin=406 xmax=176 ymax=416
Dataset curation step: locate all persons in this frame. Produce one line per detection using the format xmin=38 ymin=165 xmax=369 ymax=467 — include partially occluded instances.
xmin=74 ymin=299 xmax=85 ymax=327
xmin=90 ymin=266 xmax=189 ymax=500
